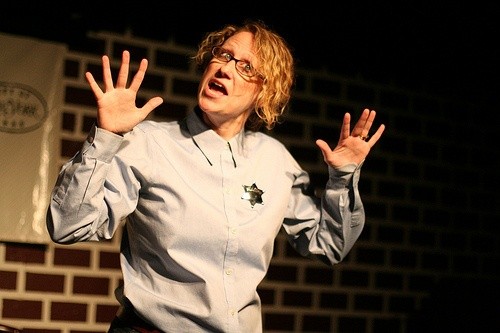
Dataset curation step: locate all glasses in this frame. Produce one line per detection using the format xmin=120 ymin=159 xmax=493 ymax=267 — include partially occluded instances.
xmin=210 ymin=44 xmax=267 ymax=83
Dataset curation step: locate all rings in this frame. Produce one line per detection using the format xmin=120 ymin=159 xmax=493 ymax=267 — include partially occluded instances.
xmin=360 ymin=135 xmax=368 ymax=140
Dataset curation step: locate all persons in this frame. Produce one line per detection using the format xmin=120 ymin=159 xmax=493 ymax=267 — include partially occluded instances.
xmin=46 ymin=21 xmax=386 ymax=333
